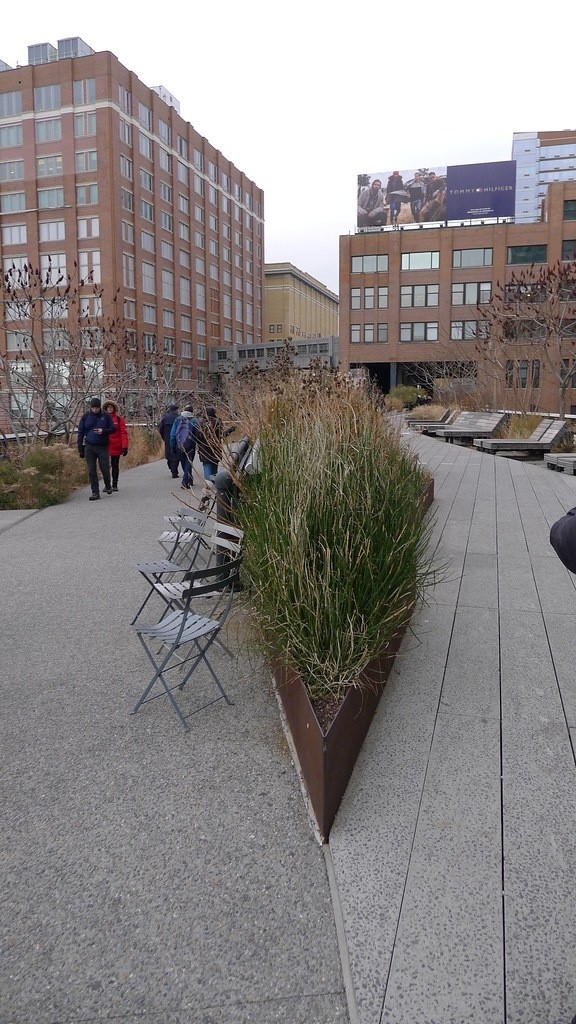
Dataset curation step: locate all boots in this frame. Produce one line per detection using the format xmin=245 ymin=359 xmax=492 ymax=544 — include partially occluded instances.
xmin=113 ymin=481 xmax=119 ymax=491
xmin=89 ymin=483 xmax=101 ymax=500
xmin=102 ymin=486 xmax=107 ymax=492
xmin=104 ymin=480 xmax=112 ymax=495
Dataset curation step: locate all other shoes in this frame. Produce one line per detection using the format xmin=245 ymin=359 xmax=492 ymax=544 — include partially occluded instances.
xmin=180 ymin=479 xmax=193 ymax=489
xmin=170 ymin=463 xmax=179 ymax=474
xmin=171 ymin=473 xmax=179 ymax=478
xmin=390 ymin=218 xmax=397 ymax=225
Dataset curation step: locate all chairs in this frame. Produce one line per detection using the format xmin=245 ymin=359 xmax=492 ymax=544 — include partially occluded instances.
xmin=542 ymin=452 xmax=576 ymax=478
xmin=126 ymin=553 xmax=244 ymax=732
xmin=127 ymin=484 xmax=215 ymax=628
xmin=472 ymin=418 xmax=567 ymax=457
xmin=402 ymin=408 xmax=505 ymax=445
xmin=149 ymin=522 xmax=248 ymax=673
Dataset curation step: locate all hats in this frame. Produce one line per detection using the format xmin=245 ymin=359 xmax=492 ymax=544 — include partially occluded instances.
xmin=170 ymin=404 xmax=180 ymax=410
xmin=90 ymin=398 xmax=102 ymax=408
xmin=387 ymin=171 xmax=403 ymax=180
xmin=204 ymin=406 xmax=216 ymax=417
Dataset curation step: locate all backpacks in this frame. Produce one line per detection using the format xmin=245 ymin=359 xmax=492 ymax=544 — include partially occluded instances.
xmin=176 ymin=417 xmax=191 ymax=451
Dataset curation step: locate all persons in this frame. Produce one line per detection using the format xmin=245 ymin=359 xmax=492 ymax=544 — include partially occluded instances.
xmin=406 ymin=172 xmax=426 ymax=222
xmin=101 ymin=398 xmax=130 ymax=493
xmin=196 ymin=405 xmax=225 ymax=484
xmin=157 ymin=404 xmax=180 ymax=480
xmin=170 ymin=404 xmax=201 ymax=490
xmin=358 ymin=179 xmax=388 ymax=226
xmin=418 ymin=171 xmax=445 ymax=223
xmin=386 ymin=171 xmax=404 ymax=223
xmin=77 ymin=397 xmax=113 ymax=501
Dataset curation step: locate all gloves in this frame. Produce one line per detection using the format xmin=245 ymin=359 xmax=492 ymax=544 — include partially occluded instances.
xmin=231 ymin=425 xmax=238 ymax=432
xmin=79 ymin=444 xmax=86 ymax=459
xmin=123 ymin=448 xmax=128 ymax=456
